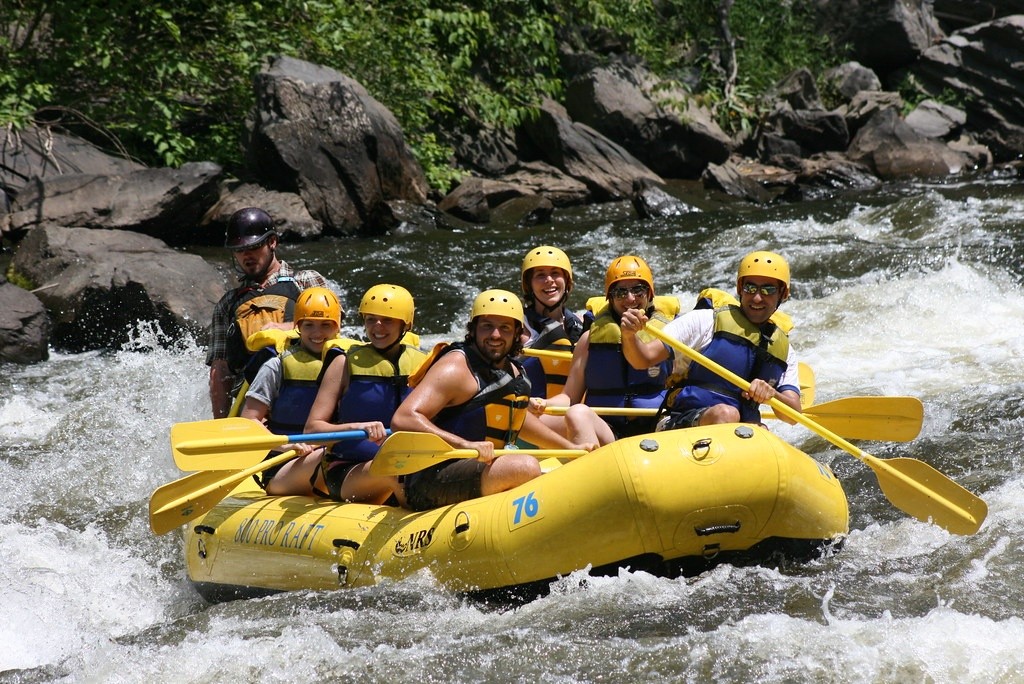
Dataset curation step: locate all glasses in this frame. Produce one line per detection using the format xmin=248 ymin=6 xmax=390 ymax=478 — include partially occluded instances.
xmin=233 ymin=237 xmax=268 ymax=252
xmin=742 ymin=281 xmax=781 ymax=296
xmin=609 ymin=284 xmax=650 ymax=301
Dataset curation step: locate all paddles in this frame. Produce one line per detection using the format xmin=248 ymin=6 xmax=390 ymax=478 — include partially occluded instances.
xmin=148 ymin=443 xmax=320 ymax=537
xmin=168 ymin=415 xmax=391 ymax=473
xmin=367 ymin=429 xmax=590 ymax=478
xmin=227 ymin=378 xmax=249 ymax=418
xmin=540 ymin=395 xmax=926 ymax=442
xmin=519 ymin=344 xmax=817 ymax=411
xmin=633 ymin=305 xmax=990 ymax=538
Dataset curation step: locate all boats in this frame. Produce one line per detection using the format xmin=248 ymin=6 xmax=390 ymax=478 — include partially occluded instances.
xmin=179 ymin=419 xmax=853 ymax=605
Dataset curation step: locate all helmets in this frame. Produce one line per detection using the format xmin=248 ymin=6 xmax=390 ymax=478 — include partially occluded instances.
xmin=521 ymin=246 xmax=573 ymax=295
xmin=224 ymin=207 xmax=279 ymax=249
xmin=293 ymin=287 xmax=341 ymax=332
xmin=358 ymin=284 xmax=414 ymax=332
xmin=472 ymin=289 xmax=524 ymax=329
xmin=606 ymin=256 xmax=654 ymax=302
xmin=736 ymin=251 xmax=790 ymax=301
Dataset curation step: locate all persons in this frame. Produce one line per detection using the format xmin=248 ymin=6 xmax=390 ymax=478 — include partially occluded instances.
xmin=390 ymin=246 xmax=584 ymax=512
xmin=528 ymin=257 xmax=675 ymax=446
xmin=240 ymin=286 xmax=341 ymax=496
xmin=622 ymin=251 xmax=803 ymax=433
xmin=205 ymin=207 xmax=329 ymax=419
xmin=303 ymin=284 xmax=414 ymax=507
